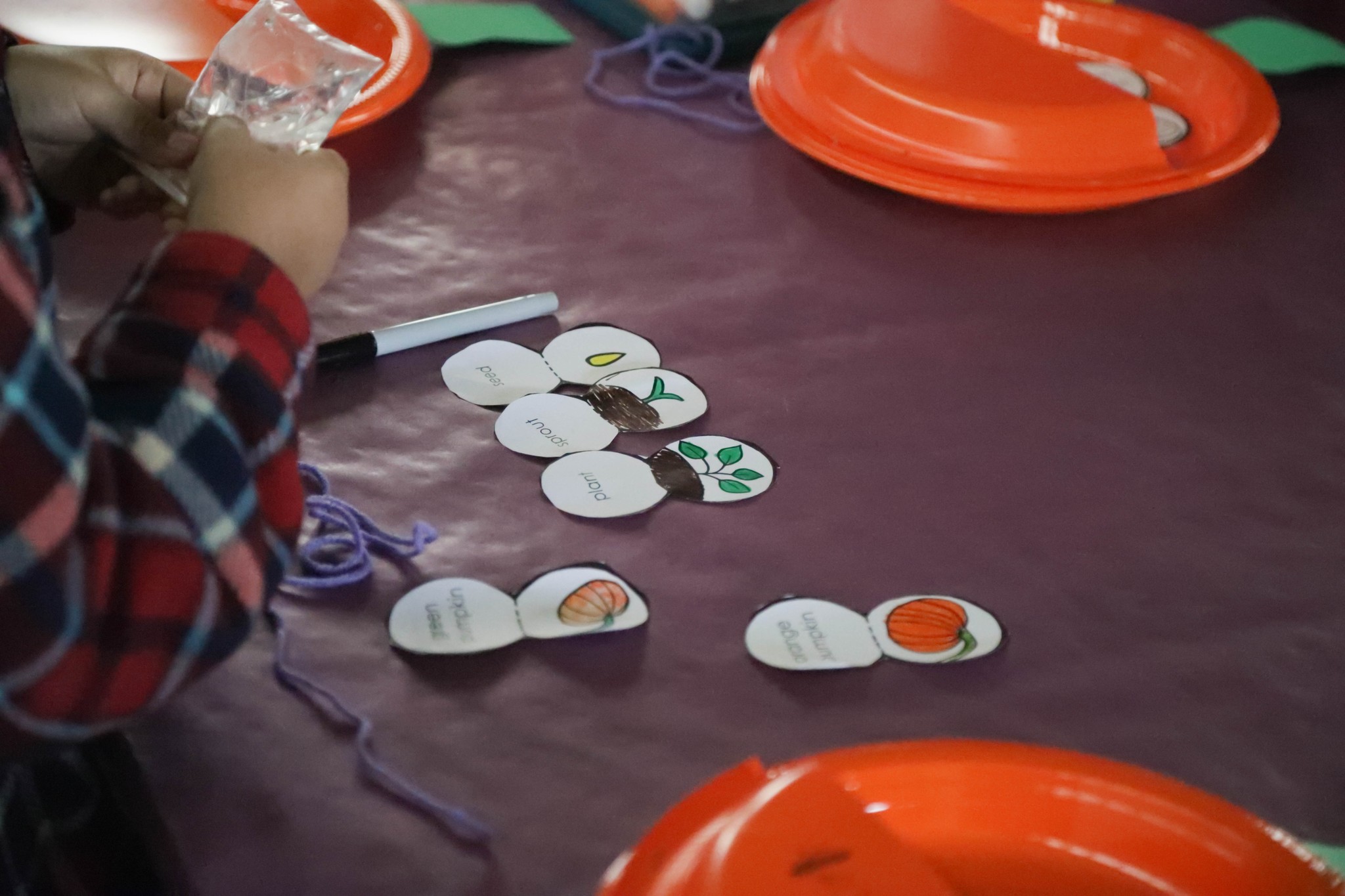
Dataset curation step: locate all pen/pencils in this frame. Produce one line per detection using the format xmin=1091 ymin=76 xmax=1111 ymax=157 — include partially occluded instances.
xmin=312 ymin=290 xmax=562 ymax=369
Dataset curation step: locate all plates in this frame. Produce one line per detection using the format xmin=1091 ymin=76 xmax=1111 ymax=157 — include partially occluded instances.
xmin=749 ymin=0 xmax=1281 ymax=214
xmin=588 ymin=737 xmax=1345 ymax=896
xmin=0 ymin=0 xmax=432 ymax=142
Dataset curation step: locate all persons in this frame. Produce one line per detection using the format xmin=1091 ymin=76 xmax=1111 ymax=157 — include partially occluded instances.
xmin=0 ymin=25 xmax=352 ymax=745
